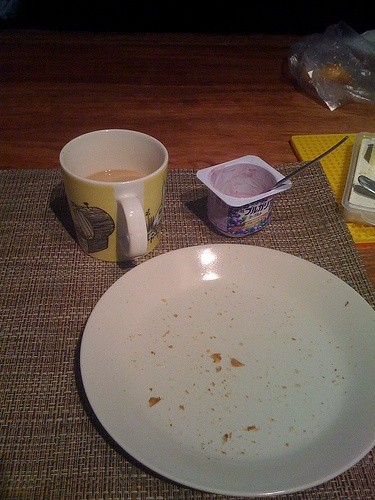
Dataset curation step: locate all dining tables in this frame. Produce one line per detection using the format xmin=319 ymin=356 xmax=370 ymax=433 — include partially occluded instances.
xmin=0 ymin=29 xmax=375 ymax=500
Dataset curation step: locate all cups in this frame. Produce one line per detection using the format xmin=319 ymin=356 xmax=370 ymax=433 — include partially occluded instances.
xmin=59 ymin=128 xmax=168 ymax=261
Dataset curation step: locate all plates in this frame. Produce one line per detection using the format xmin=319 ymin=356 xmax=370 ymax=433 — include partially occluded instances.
xmin=80 ymin=245 xmax=375 ymax=497
